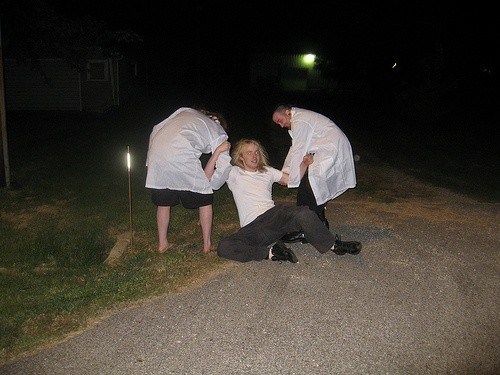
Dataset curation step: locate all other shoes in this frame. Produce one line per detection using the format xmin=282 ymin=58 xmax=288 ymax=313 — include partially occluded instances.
xmin=202 ymin=243 xmax=215 ymax=253
xmin=156 ymin=241 xmax=176 ymax=253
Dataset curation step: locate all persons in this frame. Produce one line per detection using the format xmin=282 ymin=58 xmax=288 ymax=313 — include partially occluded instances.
xmin=271 ymin=103 xmax=357 ymax=244
xmin=203 ymin=139 xmax=362 ymax=263
xmin=145 ymin=105 xmax=232 ymax=255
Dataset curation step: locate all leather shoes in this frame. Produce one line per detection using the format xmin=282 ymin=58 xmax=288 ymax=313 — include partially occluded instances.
xmin=281 ymin=231 xmax=309 ymax=243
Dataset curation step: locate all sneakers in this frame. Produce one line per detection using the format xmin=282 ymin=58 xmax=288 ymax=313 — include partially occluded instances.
xmin=330 ymin=239 xmax=362 ymax=255
xmin=271 ymin=240 xmax=298 ymax=263
